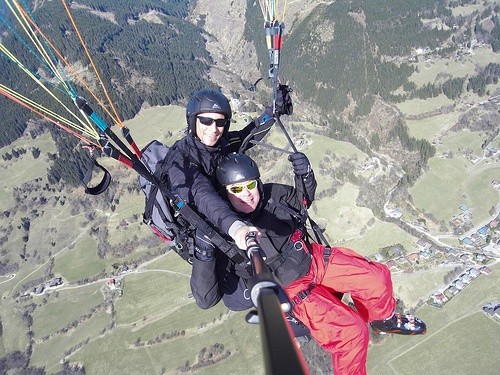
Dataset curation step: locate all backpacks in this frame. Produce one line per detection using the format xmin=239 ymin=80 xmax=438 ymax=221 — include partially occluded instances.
xmin=137 ymin=140 xmax=194 ymax=265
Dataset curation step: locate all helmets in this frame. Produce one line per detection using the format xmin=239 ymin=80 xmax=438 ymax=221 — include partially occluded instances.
xmin=186 ymin=90 xmax=232 ymax=135
xmin=216 ymin=154 xmax=264 ymax=201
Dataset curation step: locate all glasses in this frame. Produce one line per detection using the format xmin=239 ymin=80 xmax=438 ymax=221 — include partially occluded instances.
xmin=226 ymin=179 xmax=257 ymax=194
xmin=196 ymin=116 xmax=227 ymax=127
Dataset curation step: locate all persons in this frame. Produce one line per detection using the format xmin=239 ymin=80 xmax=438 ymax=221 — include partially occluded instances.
xmin=190 ymin=153 xmax=426 ymax=375
xmin=162 ymin=84 xmax=293 ymax=310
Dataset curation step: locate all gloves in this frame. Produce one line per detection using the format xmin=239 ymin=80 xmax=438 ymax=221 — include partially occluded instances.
xmin=275 ymin=84 xmax=291 ymax=106
xmin=195 ymin=220 xmax=219 ymax=263
xmin=288 ymin=152 xmax=312 ymax=181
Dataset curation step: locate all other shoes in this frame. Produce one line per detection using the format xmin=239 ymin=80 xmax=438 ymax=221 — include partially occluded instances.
xmin=370 ymin=314 xmax=426 ymax=335
